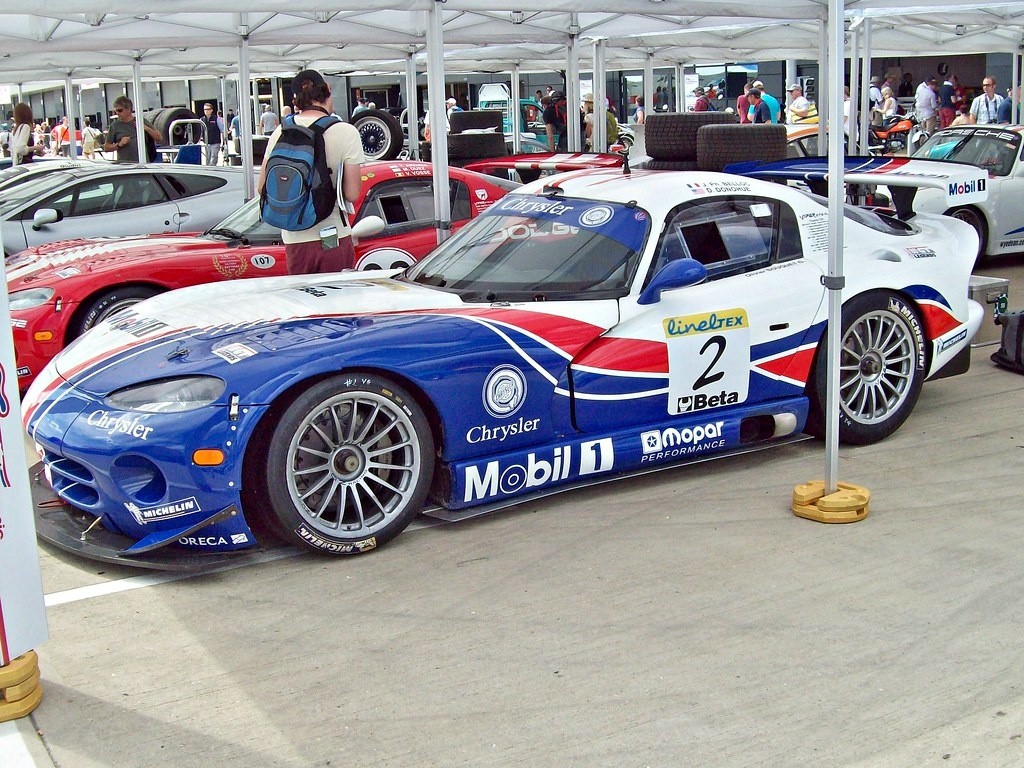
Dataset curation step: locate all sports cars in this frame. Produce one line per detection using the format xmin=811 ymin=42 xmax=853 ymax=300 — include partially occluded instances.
xmin=4 ymin=150 xmax=626 ymax=393
xmin=22 ymin=155 xmax=988 ymax=573
xmin=843 ymin=123 xmax=1024 ymax=268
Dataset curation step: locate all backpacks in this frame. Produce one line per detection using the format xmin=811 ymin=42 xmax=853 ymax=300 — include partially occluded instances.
xmin=606 ymin=111 xmax=618 ymax=144
xmin=701 ymin=95 xmax=717 ymax=111
xmin=259 ymin=112 xmax=341 ymax=232
xmin=858 ymin=86 xmax=877 ymax=111
xmin=547 ymin=96 xmax=585 ymax=128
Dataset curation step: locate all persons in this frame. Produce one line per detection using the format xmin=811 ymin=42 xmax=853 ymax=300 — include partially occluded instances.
xmin=633 ymin=78 xmax=809 ymax=124
xmin=424 ymin=86 xmax=618 ymax=154
xmin=104 ymin=96 xmax=163 ymax=191
xmin=844 ymin=72 xmax=1021 ymax=150
xmin=258 ymin=70 xmax=365 ymax=275
xmin=0 ymin=103 xmax=96 ymax=167
xmin=200 ymin=98 xmax=378 ymax=166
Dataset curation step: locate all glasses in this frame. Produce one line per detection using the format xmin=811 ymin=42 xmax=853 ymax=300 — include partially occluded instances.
xmin=114 ymin=109 xmax=123 ymax=113
xmin=982 ymin=84 xmax=993 ymax=87
xmin=204 ymin=109 xmax=210 ymax=110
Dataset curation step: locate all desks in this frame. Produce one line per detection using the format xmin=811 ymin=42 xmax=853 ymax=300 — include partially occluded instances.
xmin=155 ymin=146 xmax=208 ymax=165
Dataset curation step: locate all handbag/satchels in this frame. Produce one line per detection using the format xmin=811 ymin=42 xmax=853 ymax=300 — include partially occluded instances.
xmin=792 ymin=98 xmax=819 ymax=124
xmin=95 ymin=139 xmax=99 ymax=148
xmin=146 ymin=133 xmax=157 ymax=162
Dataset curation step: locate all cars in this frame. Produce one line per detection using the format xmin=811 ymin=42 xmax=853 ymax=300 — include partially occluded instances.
xmin=783 ymin=123 xmax=870 ymax=159
xmin=0 ymin=156 xmax=262 ymax=259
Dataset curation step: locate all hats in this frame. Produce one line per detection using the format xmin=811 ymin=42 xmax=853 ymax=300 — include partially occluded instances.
xmin=870 ymin=76 xmax=882 ymax=83
xmin=265 ymin=105 xmax=273 ymax=110
xmin=581 ymin=93 xmax=593 ymax=102
xmin=845 ymin=86 xmax=850 ymax=94
xmin=445 ymin=98 xmax=457 ymax=105
xmin=1006 ymin=80 xmax=1021 ymax=92
xmin=291 ymin=69 xmax=323 ymax=94
xmin=753 ymin=81 xmax=764 ymax=88
xmin=885 ymin=72 xmax=896 ymax=78
xmin=787 ymin=84 xmax=802 ymax=92
xmin=745 ymin=87 xmax=761 ymax=97
xmin=363 ymin=98 xmax=370 ymax=102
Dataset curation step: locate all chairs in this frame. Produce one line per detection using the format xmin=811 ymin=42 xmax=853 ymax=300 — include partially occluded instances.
xmin=175 ymin=145 xmax=201 ymax=164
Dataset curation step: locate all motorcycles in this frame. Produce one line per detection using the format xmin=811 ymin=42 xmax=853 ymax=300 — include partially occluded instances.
xmin=857 ymin=93 xmax=931 ymax=156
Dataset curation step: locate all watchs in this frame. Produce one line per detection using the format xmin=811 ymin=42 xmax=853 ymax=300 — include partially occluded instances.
xmin=116 ymin=142 xmax=122 ymax=149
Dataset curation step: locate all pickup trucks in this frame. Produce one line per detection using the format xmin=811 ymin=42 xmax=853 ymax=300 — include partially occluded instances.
xmin=475 ymin=83 xmax=559 ymax=155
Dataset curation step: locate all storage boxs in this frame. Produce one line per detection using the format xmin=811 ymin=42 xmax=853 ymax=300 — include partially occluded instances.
xmin=957 ymin=273 xmax=1011 ymax=350
